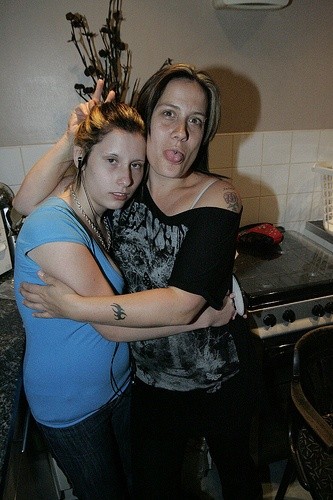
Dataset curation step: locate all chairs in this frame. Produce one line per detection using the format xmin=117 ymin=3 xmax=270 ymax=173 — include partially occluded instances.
xmin=275 ymin=325 xmax=333 ymax=500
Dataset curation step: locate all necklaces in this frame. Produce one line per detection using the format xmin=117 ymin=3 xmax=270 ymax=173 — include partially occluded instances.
xmin=65 ymin=182 xmax=112 ymax=253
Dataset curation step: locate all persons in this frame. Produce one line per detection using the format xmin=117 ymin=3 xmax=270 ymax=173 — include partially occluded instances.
xmin=12 ymin=62 xmax=262 ymax=500
xmin=13 ymin=100 xmax=239 ymax=500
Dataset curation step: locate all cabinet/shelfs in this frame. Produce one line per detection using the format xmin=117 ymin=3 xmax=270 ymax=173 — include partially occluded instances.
xmin=0 ymin=273 xmax=65 ymax=500
